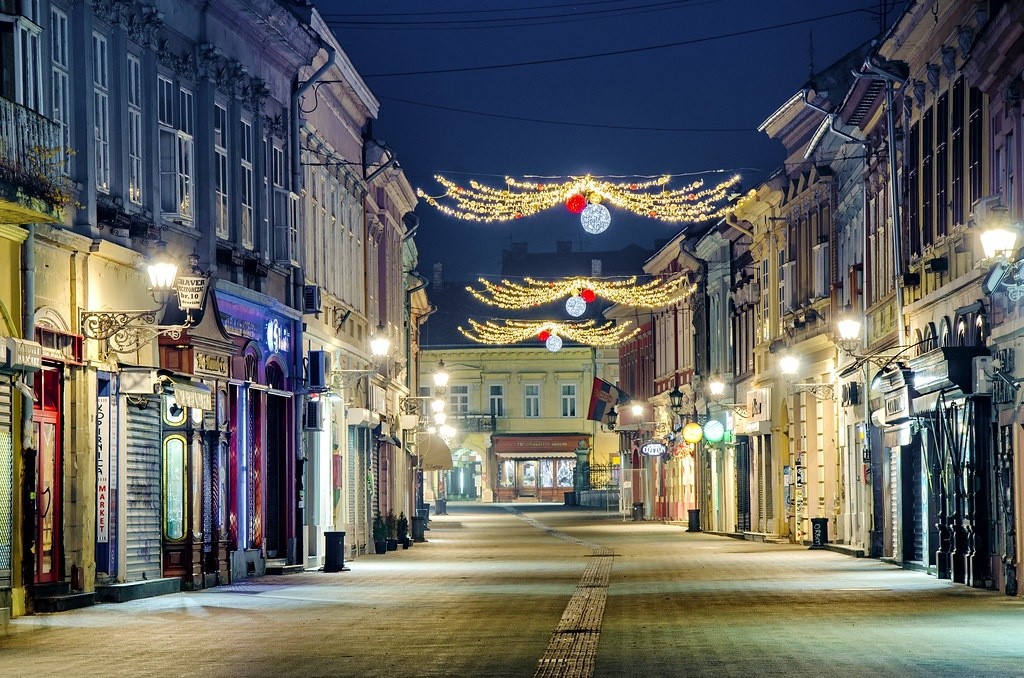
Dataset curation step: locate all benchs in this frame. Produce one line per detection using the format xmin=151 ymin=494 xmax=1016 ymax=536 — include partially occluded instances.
xmin=743 ymin=531 xmax=779 ymax=542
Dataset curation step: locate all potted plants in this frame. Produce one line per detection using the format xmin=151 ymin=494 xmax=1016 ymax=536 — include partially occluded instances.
xmin=373 ymin=508 xmax=409 ymax=555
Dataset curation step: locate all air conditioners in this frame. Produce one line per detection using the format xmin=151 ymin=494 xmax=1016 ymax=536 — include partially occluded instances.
xmin=303 ymin=284 xmax=323 ymax=314
xmin=747 ymin=283 xmax=759 ymax=305
xmin=304 ymin=401 xmax=323 ymax=432
xmin=308 ymin=350 xmax=332 ymax=390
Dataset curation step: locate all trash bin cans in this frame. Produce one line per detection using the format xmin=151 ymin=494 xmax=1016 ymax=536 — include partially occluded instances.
xmin=564 ymin=492 xmax=569 ymax=505
xmin=435 ymin=500 xmax=443 ymax=514
xmin=688 ymin=510 xmax=700 ymax=530
xmin=443 ymin=502 xmax=446 ymax=514
xmin=423 ymin=504 xmax=430 ymax=520
xmin=324 ymin=531 xmax=346 ymax=572
xmin=416 ymin=509 xmax=428 ymax=528
xmin=568 ymin=492 xmax=575 ymax=505
xmin=633 ymin=502 xmax=643 ymax=520
xmin=411 ymin=516 xmax=425 ymax=540
xmin=811 ymin=518 xmax=828 ymax=546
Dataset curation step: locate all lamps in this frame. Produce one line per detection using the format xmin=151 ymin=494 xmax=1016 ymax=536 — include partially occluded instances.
xmin=400 ymin=359 xmax=452 ymax=445
xmin=667 ymin=383 xmax=710 ymax=427
xmin=707 ymin=370 xmax=750 ymax=420
xmin=834 ymin=299 xmax=911 ymax=371
xmin=76 ymin=228 xmax=206 ymax=362
xmin=780 ymin=342 xmax=839 ymax=404
xmin=332 ymin=319 xmax=392 ymax=393
xmin=976 ymin=198 xmax=1018 ymax=286
xmin=869 ymin=335 xmax=938 ymax=391
xmin=605 ymin=402 xmax=667 ymax=440
